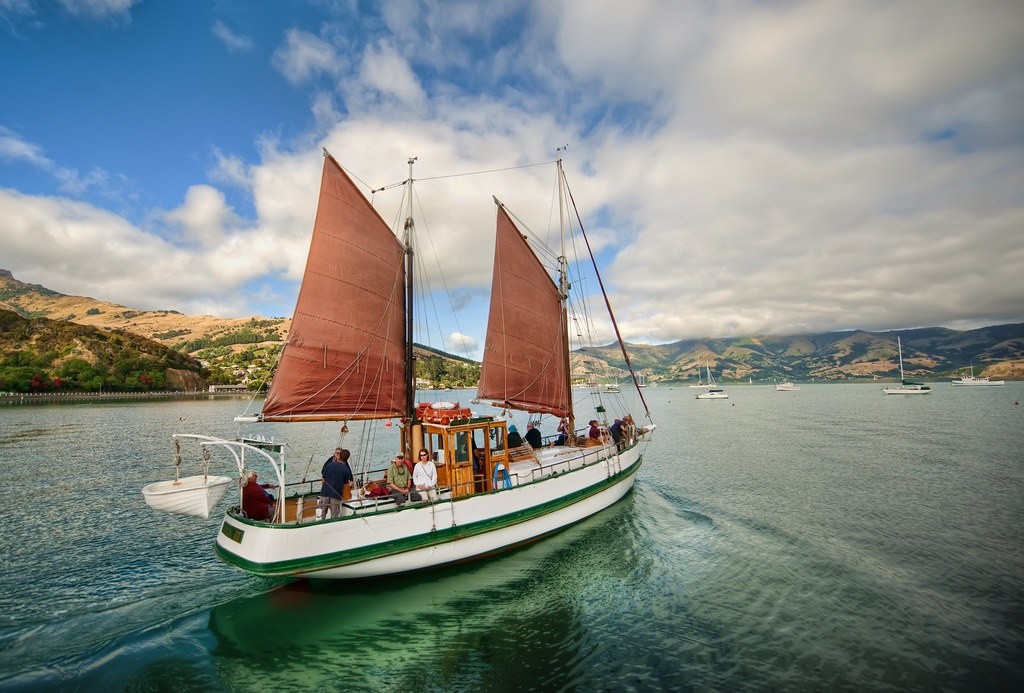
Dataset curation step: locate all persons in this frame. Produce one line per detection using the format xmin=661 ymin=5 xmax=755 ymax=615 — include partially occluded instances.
xmin=243 ymin=471 xmax=276 ymax=523
xmin=589 ymin=420 xmax=607 ymax=440
xmin=320 ymin=449 xmax=351 ymax=519
xmin=508 ymin=425 xmax=521 ymax=449
xmin=386 ymin=452 xmax=422 ymax=505
xmin=459 ymin=432 xmax=483 ymax=472
xmin=550 ymin=417 xmax=569 ymax=446
xmin=322 ymin=447 xmax=354 ymax=489
xmin=611 ymin=417 xmax=632 ymax=451
xmin=524 ymin=422 xmax=543 ymax=448
xmin=413 ymin=448 xmax=438 ymax=502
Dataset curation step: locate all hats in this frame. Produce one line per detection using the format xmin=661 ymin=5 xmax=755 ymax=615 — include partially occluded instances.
xmin=508 ymin=425 xmax=518 ymax=432
xmin=395 ymin=452 xmax=404 ymax=459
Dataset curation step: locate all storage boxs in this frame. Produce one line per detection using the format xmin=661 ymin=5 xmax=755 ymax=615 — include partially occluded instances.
xmin=425 ymin=408 xmax=473 ymax=425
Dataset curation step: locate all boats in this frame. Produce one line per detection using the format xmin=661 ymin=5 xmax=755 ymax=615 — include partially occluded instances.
xmin=695 ymin=389 xmax=728 ymax=400
xmin=142 ymin=438 xmax=232 ymax=520
xmin=950 ymin=373 xmax=1005 ymax=386
xmin=775 ymin=376 xmax=800 ymax=390
xmin=584 ymin=370 xmax=647 ymax=394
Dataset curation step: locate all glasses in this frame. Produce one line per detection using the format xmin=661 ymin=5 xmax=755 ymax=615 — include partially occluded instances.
xmin=420 ymin=454 xmax=427 ymax=456
xmin=398 ymin=457 xmax=403 ymax=459
xmin=527 ymin=426 xmax=532 ymax=427
xmin=337 ymin=452 xmax=339 ymax=454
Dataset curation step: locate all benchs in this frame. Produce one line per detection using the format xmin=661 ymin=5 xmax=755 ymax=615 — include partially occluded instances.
xmin=317 ymin=489 xmax=370 ymax=508
xmin=340 ymin=486 xmax=454 ymax=517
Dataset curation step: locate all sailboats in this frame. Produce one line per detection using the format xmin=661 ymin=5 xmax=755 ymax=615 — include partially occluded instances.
xmin=142 ymin=142 xmax=657 ymax=581
xmin=882 ymin=337 xmax=932 ymax=394
xmin=689 ymin=356 xmax=718 ymax=388
